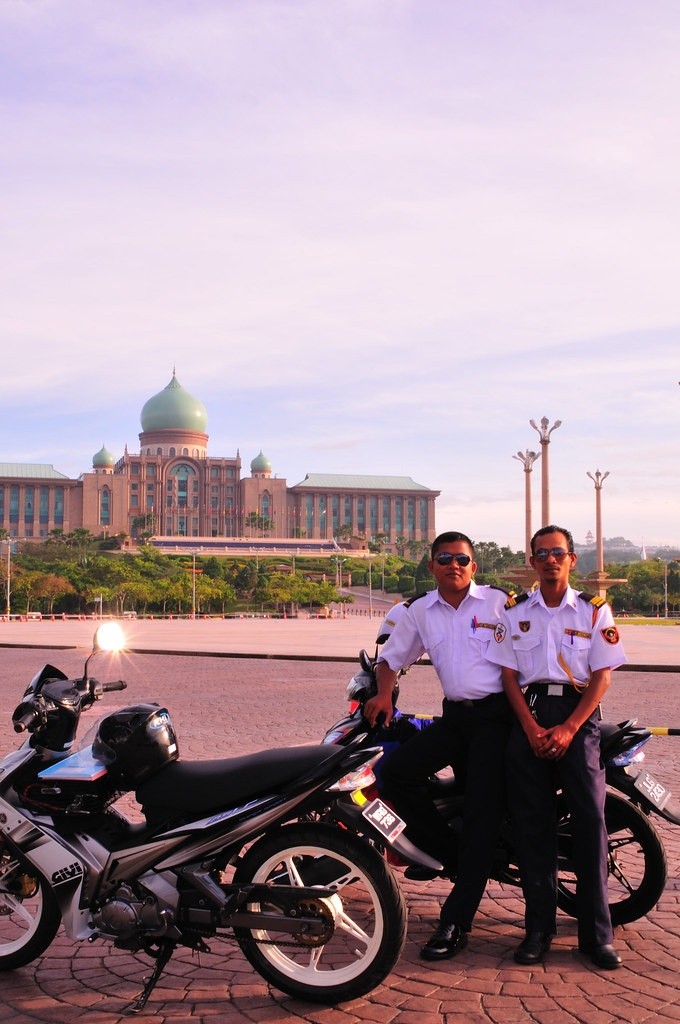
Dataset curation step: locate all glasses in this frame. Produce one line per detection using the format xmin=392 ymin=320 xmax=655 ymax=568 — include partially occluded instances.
xmin=532 ymin=549 xmax=572 ymax=561
xmin=433 ymin=552 xmax=470 ymax=567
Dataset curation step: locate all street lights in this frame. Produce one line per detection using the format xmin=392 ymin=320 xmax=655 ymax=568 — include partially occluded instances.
xmin=362 ymin=554 xmax=376 ymax=620
xmin=656 ymin=556 xmax=669 ymax=618
xmin=185 ymin=547 xmax=204 ymax=619
xmin=0 ymin=535 xmax=27 ymax=622
xmin=529 ymin=416 xmax=563 ymax=529
xmin=379 ymin=552 xmax=387 ymax=595
xmin=586 ymin=469 xmax=610 ymax=580
xmin=335 ymin=558 xmax=347 ymax=618
xmin=513 ymin=449 xmax=541 ymax=565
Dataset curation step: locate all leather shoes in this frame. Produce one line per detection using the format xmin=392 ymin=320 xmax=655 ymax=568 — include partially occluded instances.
xmin=578 ymin=932 xmax=618 ymax=969
xmin=420 ymin=922 xmax=469 ymax=961
xmin=514 ymin=929 xmax=553 ymax=965
xmin=404 ymin=859 xmax=444 ymax=882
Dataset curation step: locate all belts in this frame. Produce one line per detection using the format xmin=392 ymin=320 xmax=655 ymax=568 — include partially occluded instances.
xmin=459 ymin=698 xmax=491 ymax=709
xmin=528 ymin=682 xmax=575 ymax=697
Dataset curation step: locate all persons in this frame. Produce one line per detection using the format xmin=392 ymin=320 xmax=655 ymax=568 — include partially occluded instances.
xmin=483 ymin=525 xmax=630 ymax=968
xmin=361 ymin=532 xmax=517 ymax=962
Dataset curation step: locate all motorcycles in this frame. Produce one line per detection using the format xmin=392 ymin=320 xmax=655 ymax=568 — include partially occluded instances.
xmin=0 ymin=620 xmax=445 ymax=1011
xmin=295 ymin=631 xmax=680 ymax=929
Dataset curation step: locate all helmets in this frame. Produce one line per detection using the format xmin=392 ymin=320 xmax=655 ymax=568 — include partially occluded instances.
xmin=91 ymin=702 xmax=179 ymax=791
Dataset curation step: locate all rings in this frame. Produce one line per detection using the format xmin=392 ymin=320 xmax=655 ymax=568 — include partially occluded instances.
xmin=551 ymin=748 xmax=556 ymax=752
